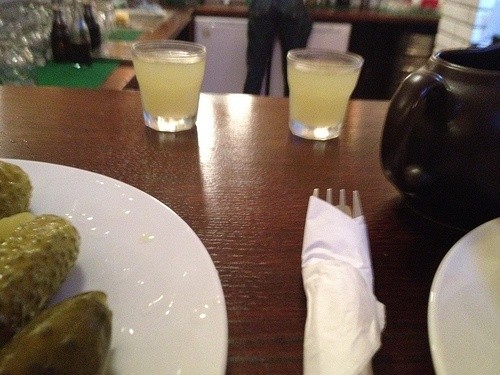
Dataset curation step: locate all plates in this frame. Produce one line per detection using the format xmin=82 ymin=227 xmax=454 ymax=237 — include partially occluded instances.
xmin=427 ymin=214 xmax=500 ymax=375
xmin=0 ymin=157 xmax=229 ymax=375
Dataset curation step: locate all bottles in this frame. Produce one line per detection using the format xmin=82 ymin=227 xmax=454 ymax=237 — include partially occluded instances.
xmin=50 ymin=3 xmax=101 ymax=65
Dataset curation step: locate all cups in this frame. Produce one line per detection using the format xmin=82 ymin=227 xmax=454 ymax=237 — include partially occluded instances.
xmin=287 ymin=47 xmax=364 ymax=140
xmin=132 ymin=39 xmax=206 ymax=132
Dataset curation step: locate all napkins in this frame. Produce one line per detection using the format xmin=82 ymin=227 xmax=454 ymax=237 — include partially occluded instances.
xmin=301 ymin=197 xmax=387 ymax=375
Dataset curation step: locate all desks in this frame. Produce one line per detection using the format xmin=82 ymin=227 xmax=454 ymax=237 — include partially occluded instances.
xmin=30 ymin=5 xmax=440 ymax=98
xmin=0 ymin=84 xmax=455 ymax=375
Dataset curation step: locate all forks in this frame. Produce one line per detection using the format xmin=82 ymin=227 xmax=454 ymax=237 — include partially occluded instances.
xmin=301 ymin=187 xmax=384 ymax=375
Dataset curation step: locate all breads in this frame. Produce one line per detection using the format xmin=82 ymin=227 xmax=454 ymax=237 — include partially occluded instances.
xmin=0 ymin=160 xmax=111 ymax=375
